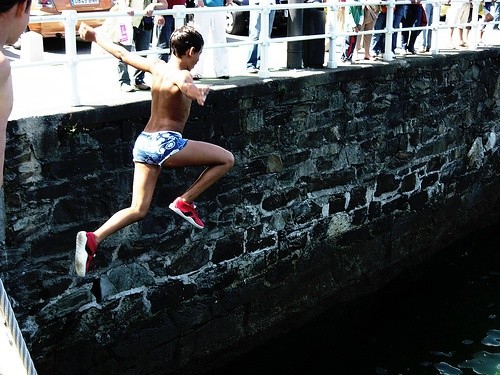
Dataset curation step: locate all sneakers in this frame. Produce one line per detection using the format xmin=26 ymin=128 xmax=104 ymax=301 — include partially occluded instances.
xmin=169 ymin=196 xmax=205 ymax=230
xmin=133 ymin=81 xmax=151 ymax=90
xmin=121 ymin=82 xmax=135 ymax=92
xmin=74 ymin=230 xmax=97 ymax=278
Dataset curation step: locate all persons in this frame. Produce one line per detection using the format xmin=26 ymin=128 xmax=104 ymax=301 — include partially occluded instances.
xmin=109 ymin=0 xmax=500 ymax=93
xmin=73 ymin=22 xmax=235 ymax=279
xmin=0 ymin=0 xmax=37 ymax=191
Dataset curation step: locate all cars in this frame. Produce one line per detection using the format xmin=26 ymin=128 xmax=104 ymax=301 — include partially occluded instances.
xmin=13 ymin=0 xmax=114 ymax=50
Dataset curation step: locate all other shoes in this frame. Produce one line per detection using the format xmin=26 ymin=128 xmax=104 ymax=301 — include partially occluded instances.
xmin=248 ymin=67 xmax=257 ymax=72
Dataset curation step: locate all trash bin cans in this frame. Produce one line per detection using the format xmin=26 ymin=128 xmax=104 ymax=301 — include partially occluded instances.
xmin=287 ymin=0 xmax=326 ymax=69
